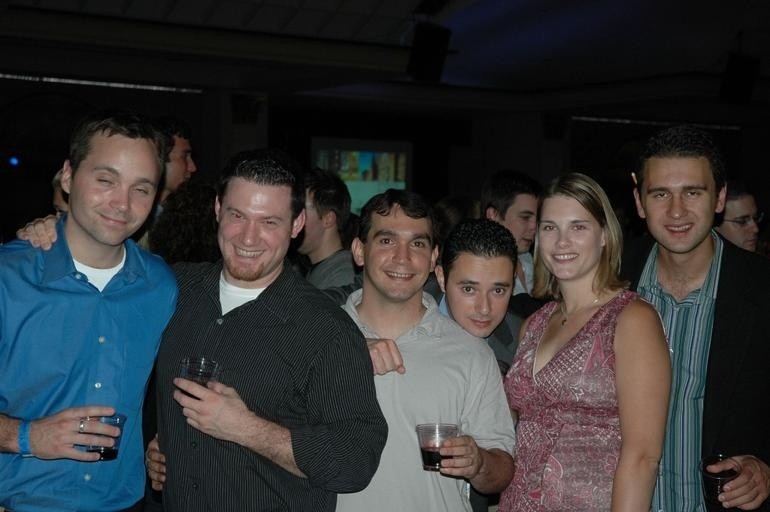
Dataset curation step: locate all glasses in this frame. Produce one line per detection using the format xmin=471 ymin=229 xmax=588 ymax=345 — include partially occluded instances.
xmin=723 ymin=212 xmax=764 ymax=226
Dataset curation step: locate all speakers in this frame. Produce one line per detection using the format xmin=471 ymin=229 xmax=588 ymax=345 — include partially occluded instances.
xmin=719 ymin=52 xmax=759 ymax=105
xmin=406 ymin=23 xmax=452 ymax=81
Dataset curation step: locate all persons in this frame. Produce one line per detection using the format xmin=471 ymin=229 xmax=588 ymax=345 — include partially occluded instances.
xmin=504 ymin=170 xmax=674 ymax=510
xmin=335 ymin=185 xmax=516 ymax=512
xmin=625 ymin=123 xmax=770 ymax=510
xmin=49 ymin=120 xmax=759 ymax=307
xmin=0 ymin=113 xmax=180 ymax=511
xmin=15 ymin=149 xmax=388 ymax=512
xmin=144 ymin=218 xmax=527 ymax=512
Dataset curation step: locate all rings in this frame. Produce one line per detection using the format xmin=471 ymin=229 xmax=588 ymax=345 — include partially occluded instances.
xmin=77 ymin=417 xmax=86 ymax=434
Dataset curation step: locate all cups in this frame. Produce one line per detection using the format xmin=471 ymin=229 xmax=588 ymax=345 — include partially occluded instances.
xmin=694 ymin=454 xmax=744 ymax=504
xmin=175 ymin=356 xmax=224 ymax=400
xmin=82 ymin=413 xmax=127 ymax=460
xmin=414 ymin=424 xmax=460 ymax=473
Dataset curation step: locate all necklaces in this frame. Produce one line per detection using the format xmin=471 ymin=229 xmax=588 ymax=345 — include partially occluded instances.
xmin=558 ymin=287 xmax=607 ymax=329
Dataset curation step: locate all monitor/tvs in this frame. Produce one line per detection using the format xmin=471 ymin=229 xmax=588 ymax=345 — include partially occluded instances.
xmin=315 ymin=136 xmax=413 ymax=216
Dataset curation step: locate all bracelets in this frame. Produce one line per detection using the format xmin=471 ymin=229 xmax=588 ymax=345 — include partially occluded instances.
xmin=16 ymin=416 xmax=34 ymax=460
